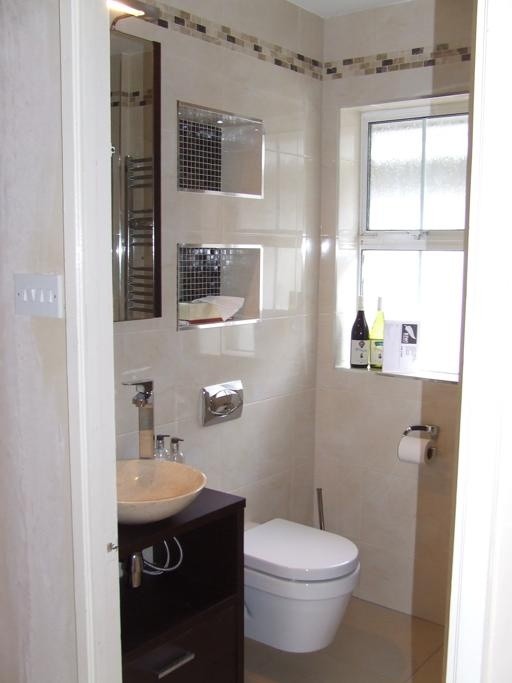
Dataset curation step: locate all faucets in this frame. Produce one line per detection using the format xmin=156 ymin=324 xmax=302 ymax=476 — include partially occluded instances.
xmin=121 ymin=379 xmax=157 ymax=460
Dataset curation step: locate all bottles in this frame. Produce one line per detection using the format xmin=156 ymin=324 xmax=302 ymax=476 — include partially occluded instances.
xmin=350 ymin=297 xmax=369 ymax=368
xmin=369 ymin=295 xmax=387 ymax=369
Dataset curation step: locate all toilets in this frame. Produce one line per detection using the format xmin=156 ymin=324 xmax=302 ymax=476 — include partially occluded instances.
xmin=243 ymin=517 xmax=361 ymax=655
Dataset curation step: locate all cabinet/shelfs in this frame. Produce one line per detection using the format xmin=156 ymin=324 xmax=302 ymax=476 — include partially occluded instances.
xmin=117 ymin=487 xmax=248 ymax=683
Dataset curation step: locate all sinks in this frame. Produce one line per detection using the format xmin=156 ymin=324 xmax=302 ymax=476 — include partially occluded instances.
xmin=117 ymin=457 xmax=208 ymax=526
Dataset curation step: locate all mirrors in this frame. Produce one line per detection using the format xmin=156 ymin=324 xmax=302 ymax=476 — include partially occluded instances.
xmin=177 ymin=100 xmax=265 ymax=200
xmin=110 ymin=27 xmax=163 ymax=322
xmin=177 ymin=243 xmax=263 ymax=331
